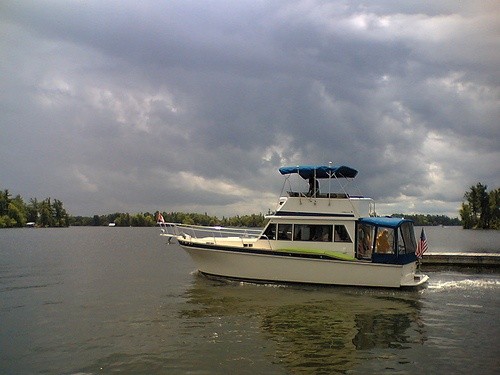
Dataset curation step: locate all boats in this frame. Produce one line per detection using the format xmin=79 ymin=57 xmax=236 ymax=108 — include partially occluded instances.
xmin=156 ymin=161 xmax=431 ymax=292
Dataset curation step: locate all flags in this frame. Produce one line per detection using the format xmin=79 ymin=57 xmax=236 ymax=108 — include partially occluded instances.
xmin=156 ymin=211 xmax=164 ymax=221
xmin=414 ymin=229 xmax=429 ymax=259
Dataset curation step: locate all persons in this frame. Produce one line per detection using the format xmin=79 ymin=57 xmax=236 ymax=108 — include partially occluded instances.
xmin=280 ymin=230 xmax=341 ymax=241
xmin=305 ymin=175 xmax=319 ymax=196
xmin=375 ymin=229 xmax=392 ymax=253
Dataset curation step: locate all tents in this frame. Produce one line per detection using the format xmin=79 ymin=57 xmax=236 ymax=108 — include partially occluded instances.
xmin=279 ymin=166 xmax=359 ymax=198
